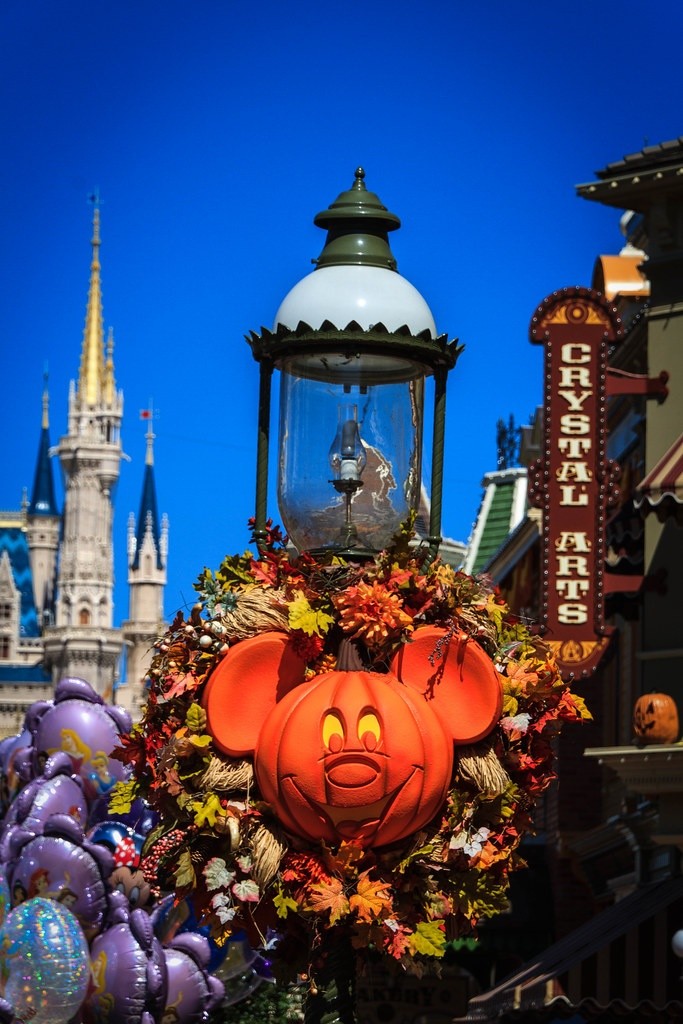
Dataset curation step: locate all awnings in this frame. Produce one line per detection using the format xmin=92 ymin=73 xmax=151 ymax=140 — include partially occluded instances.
xmin=465 ymin=876 xmax=683 ymax=1024
xmin=632 ymin=434 xmax=683 ymax=522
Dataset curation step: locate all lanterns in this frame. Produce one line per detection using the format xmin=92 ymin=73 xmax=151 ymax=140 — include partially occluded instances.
xmin=203 ymin=625 xmax=504 ymax=849
xmin=633 ymin=692 xmax=679 ymax=745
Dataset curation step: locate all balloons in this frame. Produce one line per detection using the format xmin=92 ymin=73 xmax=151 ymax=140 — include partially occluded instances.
xmin=0 ymin=677 xmax=225 ymax=1024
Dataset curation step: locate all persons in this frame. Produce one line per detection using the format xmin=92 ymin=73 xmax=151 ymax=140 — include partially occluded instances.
xmin=554 ymin=830 xmax=609 ymax=942
xmin=361 ymin=945 xmax=399 ymax=1001
xmin=258 ymin=937 xmax=302 ymax=999
xmin=438 ymin=948 xmax=478 ymax=994
